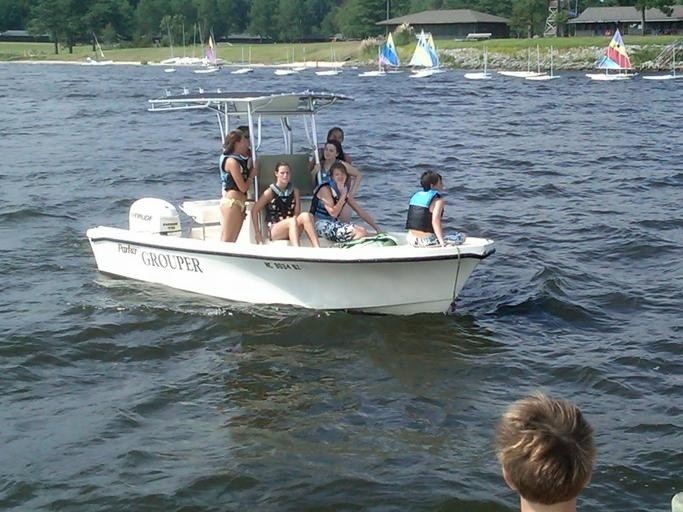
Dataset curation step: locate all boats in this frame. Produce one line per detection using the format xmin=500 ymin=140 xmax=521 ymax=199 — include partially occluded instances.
xmin=81 ymin=57 xmax=113 ymax=66
xmin=86 ymin=87 xmax=496 ymax=316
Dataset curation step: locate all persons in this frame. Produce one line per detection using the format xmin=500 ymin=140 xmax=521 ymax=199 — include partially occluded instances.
xmin=491 ymin=386 xmax=596 ymax=512
xmin=403 ymin=169 xmax=467 ymax=249
xmin=215 ymin=125 xmax=385 ymax=254
